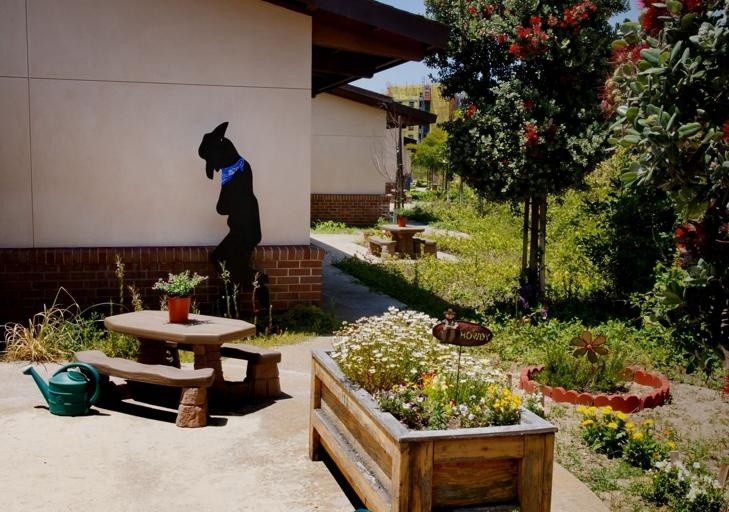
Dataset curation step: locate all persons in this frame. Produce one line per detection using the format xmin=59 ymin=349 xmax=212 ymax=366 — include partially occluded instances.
xmin=196 ymin=120 xmax=271 ymax=323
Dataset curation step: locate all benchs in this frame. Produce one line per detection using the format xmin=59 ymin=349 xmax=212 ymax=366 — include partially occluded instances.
xmin=368 ymin=235 xmax=437 ymax=258
xmin=73 ymin=342 xmax=282 ymax=428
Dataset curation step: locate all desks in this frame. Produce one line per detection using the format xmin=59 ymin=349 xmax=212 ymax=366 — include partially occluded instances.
xmin=103 ymin=310 xmax=257 ymax=405
xmin=382 ymin=224 xmax=425 ymax=259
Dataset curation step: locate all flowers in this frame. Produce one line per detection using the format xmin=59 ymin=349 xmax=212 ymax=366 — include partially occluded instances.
xmin=330 ymin=304 xmax=524 ymax=430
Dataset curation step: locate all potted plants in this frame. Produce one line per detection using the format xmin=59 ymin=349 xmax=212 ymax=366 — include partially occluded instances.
xmin=393 ymin=208 xmax=416 ymax=227
xmin=151 ymin=268 xmax=209 ymax=323
